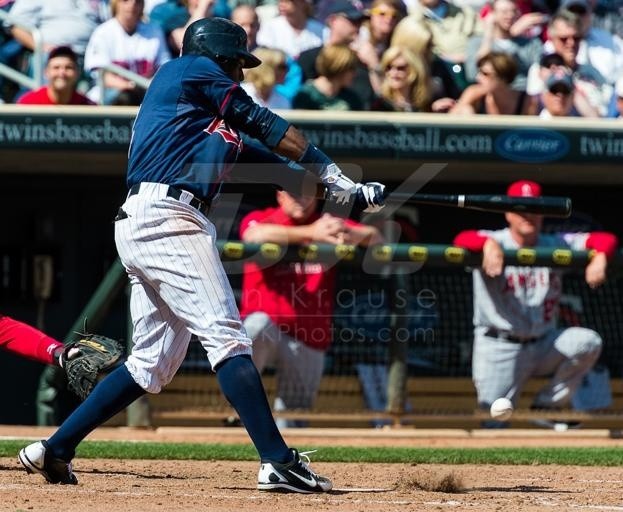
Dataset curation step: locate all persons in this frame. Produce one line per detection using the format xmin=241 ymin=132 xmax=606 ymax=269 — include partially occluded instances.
xmin=454 ymin=181 xmax=616 ymax=432
xmin=13 ymin=17 xmax=387 ymax=493
xmin=236 ymin=182 xmax=381 ymax=432
xmin=1 ymin=0 xmax=622 ymax=116
xmin=0 ymin=317 xmax=125 ymax=400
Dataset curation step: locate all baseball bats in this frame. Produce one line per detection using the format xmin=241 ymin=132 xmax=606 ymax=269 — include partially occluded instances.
xmin=323 ymin=188 xmax=571 ymax=217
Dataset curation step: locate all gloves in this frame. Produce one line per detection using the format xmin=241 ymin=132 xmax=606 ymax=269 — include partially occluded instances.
xmin=319 ymin=162 xmax=357 ymax=205
xmin=355 ymin=181 xmax=389 ymax=214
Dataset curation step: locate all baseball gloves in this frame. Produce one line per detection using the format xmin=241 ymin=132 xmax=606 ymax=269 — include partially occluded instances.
xmin=63 ymin=330 xmax=123 ymax=400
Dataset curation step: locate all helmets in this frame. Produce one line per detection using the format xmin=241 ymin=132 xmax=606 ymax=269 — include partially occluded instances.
xmin=179 ymin=16 xmax=262 ymax=70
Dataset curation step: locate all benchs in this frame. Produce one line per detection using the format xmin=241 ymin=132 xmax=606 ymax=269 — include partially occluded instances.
xmin=92 ymin=357 xmax=623 ymax=428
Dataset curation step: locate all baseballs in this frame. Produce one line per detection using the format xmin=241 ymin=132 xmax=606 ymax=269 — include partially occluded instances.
xmin=491 ymin=399 xmax=513 ymax=421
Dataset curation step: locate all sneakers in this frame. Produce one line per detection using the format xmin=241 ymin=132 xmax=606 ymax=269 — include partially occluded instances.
xmin=255 ymin=446 xmax=335 ymax=494
xmin=530 ymin=403 xmax=584 ymax=430
xmin=17 ymin=439 xmax=79 ymax=485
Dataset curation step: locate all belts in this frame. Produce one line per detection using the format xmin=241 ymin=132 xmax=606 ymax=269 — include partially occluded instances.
xmin=476 ymin=325 xmax=540 ymax=346
xmin=128 ymin=184 xmax=211 ymax=215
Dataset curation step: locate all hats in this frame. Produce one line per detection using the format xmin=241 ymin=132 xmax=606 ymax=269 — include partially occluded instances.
xmin=46 ymin=46 xmax=80 ymax=60
xmin=319 ymin=0 xmax=373 ymax=21
xmin=541 ymin=70 xmax=574 ymax=90
xmin=506 ymin=181 xmax=542 ymax=200
